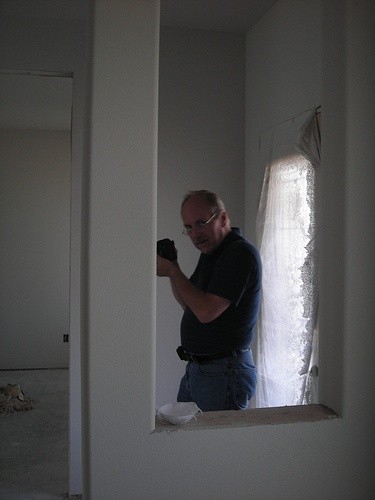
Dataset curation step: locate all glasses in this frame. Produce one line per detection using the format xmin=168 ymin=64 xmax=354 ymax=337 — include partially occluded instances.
xmin=182 ymin=213 xmax=217 ymax=236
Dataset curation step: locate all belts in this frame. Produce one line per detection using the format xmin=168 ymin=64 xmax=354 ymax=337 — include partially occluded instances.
xmin=183 ymin=346 xmax=251 ymax=364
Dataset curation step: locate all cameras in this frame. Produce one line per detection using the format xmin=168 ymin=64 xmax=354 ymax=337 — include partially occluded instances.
xmin=157 ymin=237 xmax=177 ymax=262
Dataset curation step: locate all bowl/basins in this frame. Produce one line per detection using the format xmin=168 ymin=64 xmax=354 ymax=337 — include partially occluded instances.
xmin=157 ymin=402 xmax=196 ymax=426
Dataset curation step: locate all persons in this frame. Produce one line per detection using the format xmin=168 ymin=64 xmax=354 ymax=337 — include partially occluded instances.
xmin=157 ymin=189 xmax=263 ymax=412
